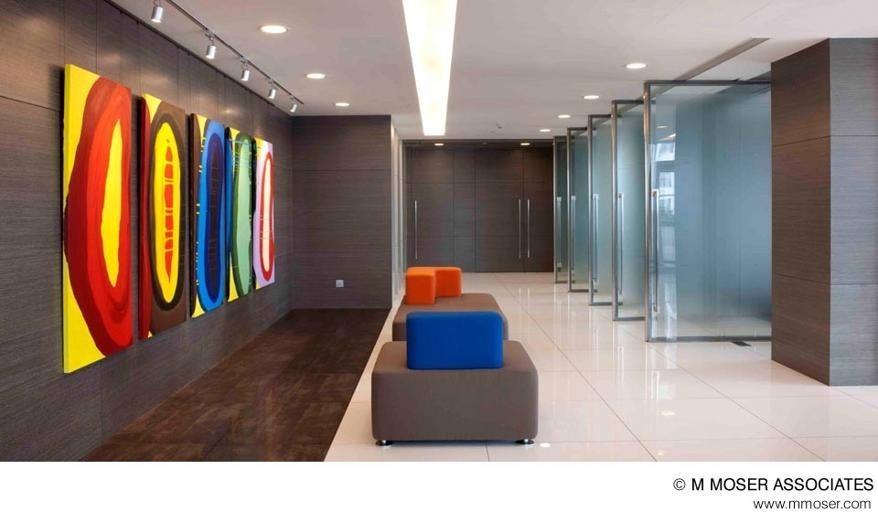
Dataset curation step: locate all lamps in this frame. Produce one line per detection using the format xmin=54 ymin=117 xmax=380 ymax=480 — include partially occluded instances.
xmin=150 ymin=0 xmax=305 ymax=114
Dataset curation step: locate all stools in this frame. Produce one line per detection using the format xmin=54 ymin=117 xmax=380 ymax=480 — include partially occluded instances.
xmin=370 ymin=264 xmax=539 ymax=446
